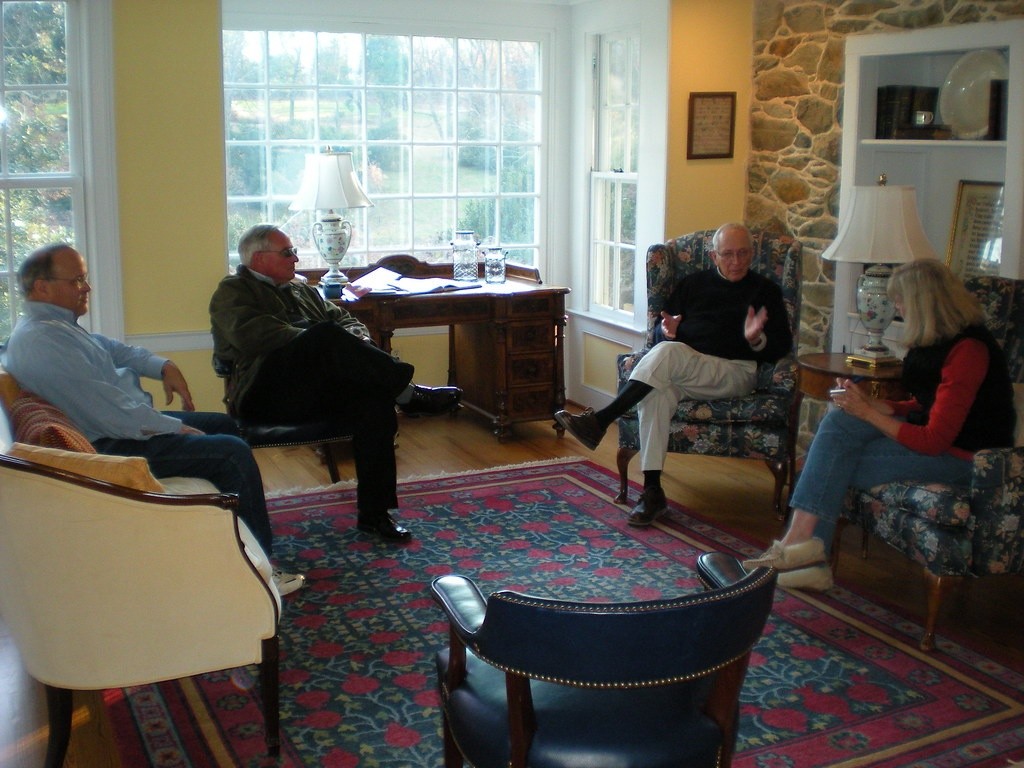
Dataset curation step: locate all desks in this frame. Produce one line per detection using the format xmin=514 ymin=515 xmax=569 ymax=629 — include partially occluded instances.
xmin=296 ymin=252 xmax=573 ymax=466
xmin=785 ymin=348 xmax=911 ymax=557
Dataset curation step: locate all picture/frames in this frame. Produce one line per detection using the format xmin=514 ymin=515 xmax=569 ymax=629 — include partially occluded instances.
xmin=832 ymin=20 xmax=1024 ymax=358
xmin=944 ymin=178 xmax=1004 ymax=269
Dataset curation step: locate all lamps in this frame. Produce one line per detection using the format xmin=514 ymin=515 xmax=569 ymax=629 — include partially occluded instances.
xmin=288 ymin=142 xmax=376 ymax=289
xmin=820 ymin=172 xmax=940 ymax=368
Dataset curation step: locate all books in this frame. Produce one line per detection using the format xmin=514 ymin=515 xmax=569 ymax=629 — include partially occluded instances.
xmin=989 ymin=79 xmax=1007 ymax=140
xmin=876 ymin=85 xmax=953 ymax=141
xmin=342 ymin=266 xmax=482 ymax=300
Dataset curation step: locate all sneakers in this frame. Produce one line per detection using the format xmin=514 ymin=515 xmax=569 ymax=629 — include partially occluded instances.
xmin=772 ymin=562 xmax=834 ymax=592
xmin=739 ymin=534 xmax=828 ymax=570
xmin=271 ymin=564 xmax=303 ymax=597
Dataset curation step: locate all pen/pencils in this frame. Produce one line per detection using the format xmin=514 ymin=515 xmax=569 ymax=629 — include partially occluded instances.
xmin=841 ymin=375 xmax=864 ymax=389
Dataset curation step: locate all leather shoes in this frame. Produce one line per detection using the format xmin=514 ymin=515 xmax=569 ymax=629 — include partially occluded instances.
xmin=554 ymin=405 xmax=607 ymax=453
xmin=397 ymin=382 xmax=464 ymax=419
xmin=358 ymin=508 xmax=411 ymax=542
xmin=625 ymin=485 xmax=669 ymax=526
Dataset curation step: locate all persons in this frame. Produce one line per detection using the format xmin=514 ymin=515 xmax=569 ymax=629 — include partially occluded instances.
xmin=0 ymin=243 xmax=306 ymax=596
xmin=554 ymin=223 xmax=795 ymax=524
xmin=743 ymin=258 xmax=1016 ymax=591
xmin=208 ymin=223 xmax=464 ymax=541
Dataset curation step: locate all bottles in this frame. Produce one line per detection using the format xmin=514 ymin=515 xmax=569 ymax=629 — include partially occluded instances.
xmin=452 ymin=230 xmax=479 ymax=282
xmin=484 ymin=245 xmax=507 ymax=284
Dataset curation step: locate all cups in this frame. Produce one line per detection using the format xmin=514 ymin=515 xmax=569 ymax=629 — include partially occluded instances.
xmin=322 ymin=282 xmax=345 ymax=299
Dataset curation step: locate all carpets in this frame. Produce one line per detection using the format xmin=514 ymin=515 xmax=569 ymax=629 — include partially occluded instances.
xmin=100 ymin=454 xmax=1024 ymax=768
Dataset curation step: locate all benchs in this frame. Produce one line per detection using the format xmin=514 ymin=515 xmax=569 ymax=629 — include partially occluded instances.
xmin=0 ymin=345 xmax=287 ymax=768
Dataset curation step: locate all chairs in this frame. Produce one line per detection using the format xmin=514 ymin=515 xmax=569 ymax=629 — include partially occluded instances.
xmin=429 ymin=546 xmax=781 ymax=768
xmin=615 ymin=222 xmax=806 ymax=518
xmin=816 ymin=273 xmax=1024 ymax=650
xmin=211 ymin=338 xmax=368 ymax=484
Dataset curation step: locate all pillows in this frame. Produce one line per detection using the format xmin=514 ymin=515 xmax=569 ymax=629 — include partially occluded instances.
xmin=7 ymin=394 xmax=99 ymax=456
xmin=7 ymin=439 xmax=169 ymax=494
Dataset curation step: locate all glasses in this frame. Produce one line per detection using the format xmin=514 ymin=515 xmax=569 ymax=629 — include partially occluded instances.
xmin=253 ymin=247 xmax=297 ymax=259
xmin=45 ymin=272 xmax=89 ymax=288
xmin=716 ymin=249 xmax=753 ymax=260
